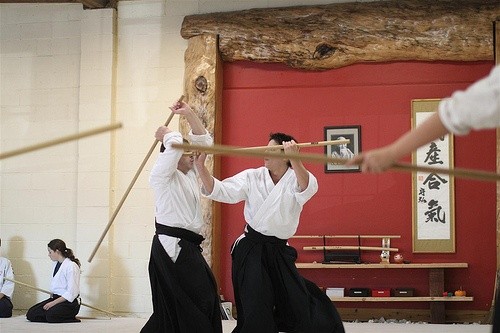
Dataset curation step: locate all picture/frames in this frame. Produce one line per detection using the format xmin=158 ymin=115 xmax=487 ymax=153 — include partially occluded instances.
xmin=325 ymin=124 xmax=363 ymax=173
xmin=410 ymin=98 xmax=456 ymax=255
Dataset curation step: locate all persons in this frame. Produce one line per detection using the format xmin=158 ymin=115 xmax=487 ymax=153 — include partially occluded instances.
xmin=191 ymin=132 xmax=346 ymax=333
xmin=0 ymin=238 xmax=15 ymax=318
xmin=343 ymin=63 xmax=500 ymax=333
xmin=25 ymin=238 xmax=82 ymax=323
xmin=331 ymin=137 xmax=354 ymax=165
xmin=137 ymin=101 xmax=223 ymax=333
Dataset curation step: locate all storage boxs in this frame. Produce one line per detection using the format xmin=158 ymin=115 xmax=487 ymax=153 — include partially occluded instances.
xmin=319 ymin=287 xmax=412 ymax=297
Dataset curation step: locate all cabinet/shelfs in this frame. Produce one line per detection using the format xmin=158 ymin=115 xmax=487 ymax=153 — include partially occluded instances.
xmin=294 ymin=262 xmax=475 ymax=322
